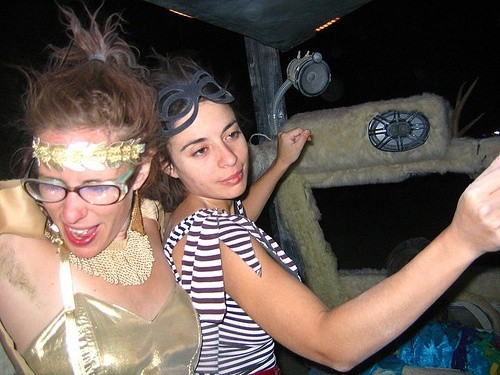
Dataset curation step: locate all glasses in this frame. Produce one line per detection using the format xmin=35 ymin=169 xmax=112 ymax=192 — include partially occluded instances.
xmin=22 ymin=159 xmax=142 ymax=207
